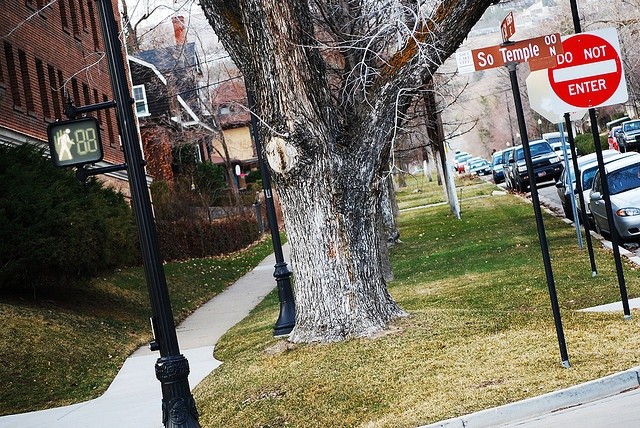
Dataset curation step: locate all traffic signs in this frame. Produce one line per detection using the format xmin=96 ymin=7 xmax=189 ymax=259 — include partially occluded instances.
xmin=499 ymin=11 xmax=515 ymax=43
xmin=470 ymin=32 xmax=565 ymax=73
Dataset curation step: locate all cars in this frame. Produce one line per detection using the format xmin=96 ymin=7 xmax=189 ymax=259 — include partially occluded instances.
xmin=500 ymin=146 xmax=515 ymax=189
xmin=555 ymin=149 xmax=622 ymax=223
xmin=550 ymin=142 xmax=578 ymax=160
xmin=490 ymin=153 xmax=505 ymax=185
xmin=572 ymin=150 xmax=639 ymax=230
xmin=589 ymin=153 xmax=640 ymax=250
xmin=453 ymin=149 xmax=492 ymax=176
xmin=608 ymin=126 xmax=623 ymax=147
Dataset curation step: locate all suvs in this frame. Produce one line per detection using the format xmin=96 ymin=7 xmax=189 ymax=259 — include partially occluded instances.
xmin=508 ymin=139 xmax=564 ymax=194
xmin=616 ymin=119 xmax=640 ymax=153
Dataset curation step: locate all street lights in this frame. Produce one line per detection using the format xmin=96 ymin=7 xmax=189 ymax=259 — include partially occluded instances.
xmin=538 ymin=118 xmax=543 ymax=139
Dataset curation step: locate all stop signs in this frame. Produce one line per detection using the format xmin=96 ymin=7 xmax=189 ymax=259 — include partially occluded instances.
xmin=546 ymin=25 xmax=628 ymax=114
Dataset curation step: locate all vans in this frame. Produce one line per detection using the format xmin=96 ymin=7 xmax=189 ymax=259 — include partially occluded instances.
xmin=606 ymin=116 xmax=632 ymax=131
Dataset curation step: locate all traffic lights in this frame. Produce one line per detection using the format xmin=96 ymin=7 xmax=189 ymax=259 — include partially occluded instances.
xmin=46 ymin=115 xmax=103 ymax=169
